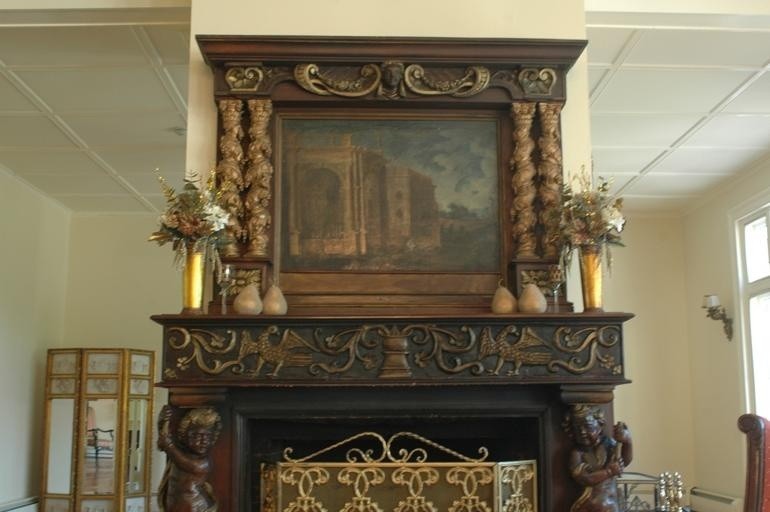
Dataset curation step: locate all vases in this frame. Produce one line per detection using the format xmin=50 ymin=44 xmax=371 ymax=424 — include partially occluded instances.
xmin=579 ymin=245 xmax=602 ymax=314
xmin=180 ymin=240 xmax=208 ymax=313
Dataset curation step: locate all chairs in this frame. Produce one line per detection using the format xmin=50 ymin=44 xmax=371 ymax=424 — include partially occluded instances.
xmin=738 ymin=413 xmax=770 ymax=512
xmin=84 ymin=408 xmax=114 ymax=458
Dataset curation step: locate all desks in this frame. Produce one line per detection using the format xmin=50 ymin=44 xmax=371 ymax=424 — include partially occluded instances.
xmin=615 ymin=470 xmax=659 ymax=510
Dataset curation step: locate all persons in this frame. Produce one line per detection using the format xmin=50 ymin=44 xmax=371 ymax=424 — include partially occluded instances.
xmin=560 ymin=402 xmax=635 ymax=511
xmin=154 ymin=403 xmax=225 ymax=511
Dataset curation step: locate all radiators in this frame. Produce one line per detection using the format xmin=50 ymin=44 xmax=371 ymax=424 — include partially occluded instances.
xmin=686 ymin=486 xmax=744 ymax=512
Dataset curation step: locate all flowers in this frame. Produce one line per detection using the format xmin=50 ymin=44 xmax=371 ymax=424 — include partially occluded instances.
xmin=148 ymin=169 xmax=233 ymax=264
xmin=547 ymin=166 xmax=625 ymax=276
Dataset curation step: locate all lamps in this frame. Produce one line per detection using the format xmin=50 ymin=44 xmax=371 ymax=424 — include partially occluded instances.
xmin=700 ymin=293 xmax=732 ymax=342
